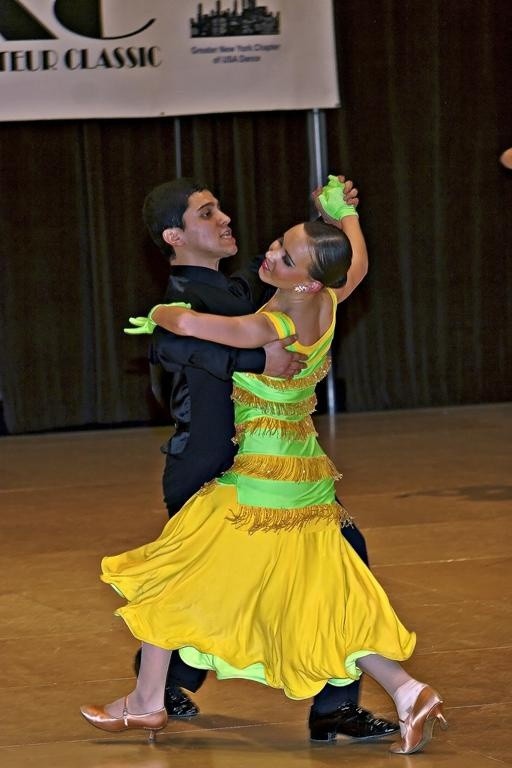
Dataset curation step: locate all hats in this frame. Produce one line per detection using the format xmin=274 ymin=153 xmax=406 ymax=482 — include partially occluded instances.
xmin=80 ymin=695 xmax=168 ymax=741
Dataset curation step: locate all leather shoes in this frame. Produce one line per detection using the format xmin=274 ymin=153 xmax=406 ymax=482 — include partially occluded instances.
xmin=163 ymin=686 xmax=200 ymax=718
xmin=306 ymin=703 xmax=399 ymax=744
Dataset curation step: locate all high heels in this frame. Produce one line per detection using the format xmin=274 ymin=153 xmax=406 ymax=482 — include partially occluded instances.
xmin=388 ymin=684 xmax=451 ymax=754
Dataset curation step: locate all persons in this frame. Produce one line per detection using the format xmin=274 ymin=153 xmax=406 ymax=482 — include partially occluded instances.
xmin=133 ymin=174 xmax=400 ymax=750
xmin=76 ymin=175 xmax=449 ymax=755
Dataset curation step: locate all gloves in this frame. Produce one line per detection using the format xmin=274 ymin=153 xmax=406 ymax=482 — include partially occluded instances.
xmin=317 ymin=173 xmax=360 ymax=221
xmin=123 ymin=301 xmax=192 ymax=336
